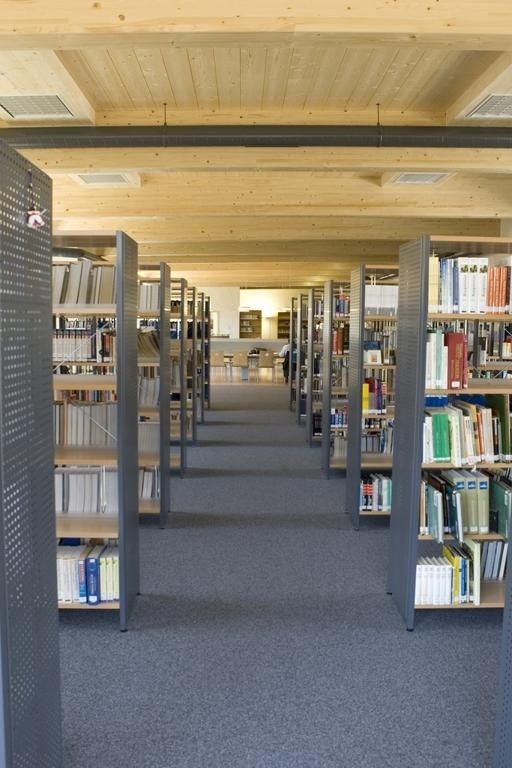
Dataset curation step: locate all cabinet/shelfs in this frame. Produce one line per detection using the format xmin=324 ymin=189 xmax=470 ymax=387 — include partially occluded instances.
xmin=240 ymin=309 xmax=263 ymax=339
xmin=277 ymin=311 xmax=290 ymax=339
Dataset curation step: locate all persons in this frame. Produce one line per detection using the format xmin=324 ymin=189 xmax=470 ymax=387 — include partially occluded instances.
xmin=278 ymin=338 xmax=290 ymax=383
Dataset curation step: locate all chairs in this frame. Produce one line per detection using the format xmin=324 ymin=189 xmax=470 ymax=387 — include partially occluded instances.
xmin=256 ymin=351 xmax=275 ymax=383
xmin=229 ymin=348 xmax=250 ymax=383
xmin=210 ymin=349 xmax=228 ymax=382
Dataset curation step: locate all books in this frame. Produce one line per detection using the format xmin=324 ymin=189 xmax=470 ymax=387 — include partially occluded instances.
xmin=139 ymin=282 xmax=162 ymax=502
xmin=364 ymin=281 xmax=399 ymax=515
xmin=293 ymin=300 xmax=324 ymax=435
xmin=414 ymin=256 xmax=511 ymax=608
xmin=241 ymin=314 xmax=257 ymax=332
xmin=54 ymin=259 xmax=120 ymax=603
xmin=329 ymin=282 xmax=351 ymax=468
xmin=168 ymin=301 xmax=209 ymax=467
